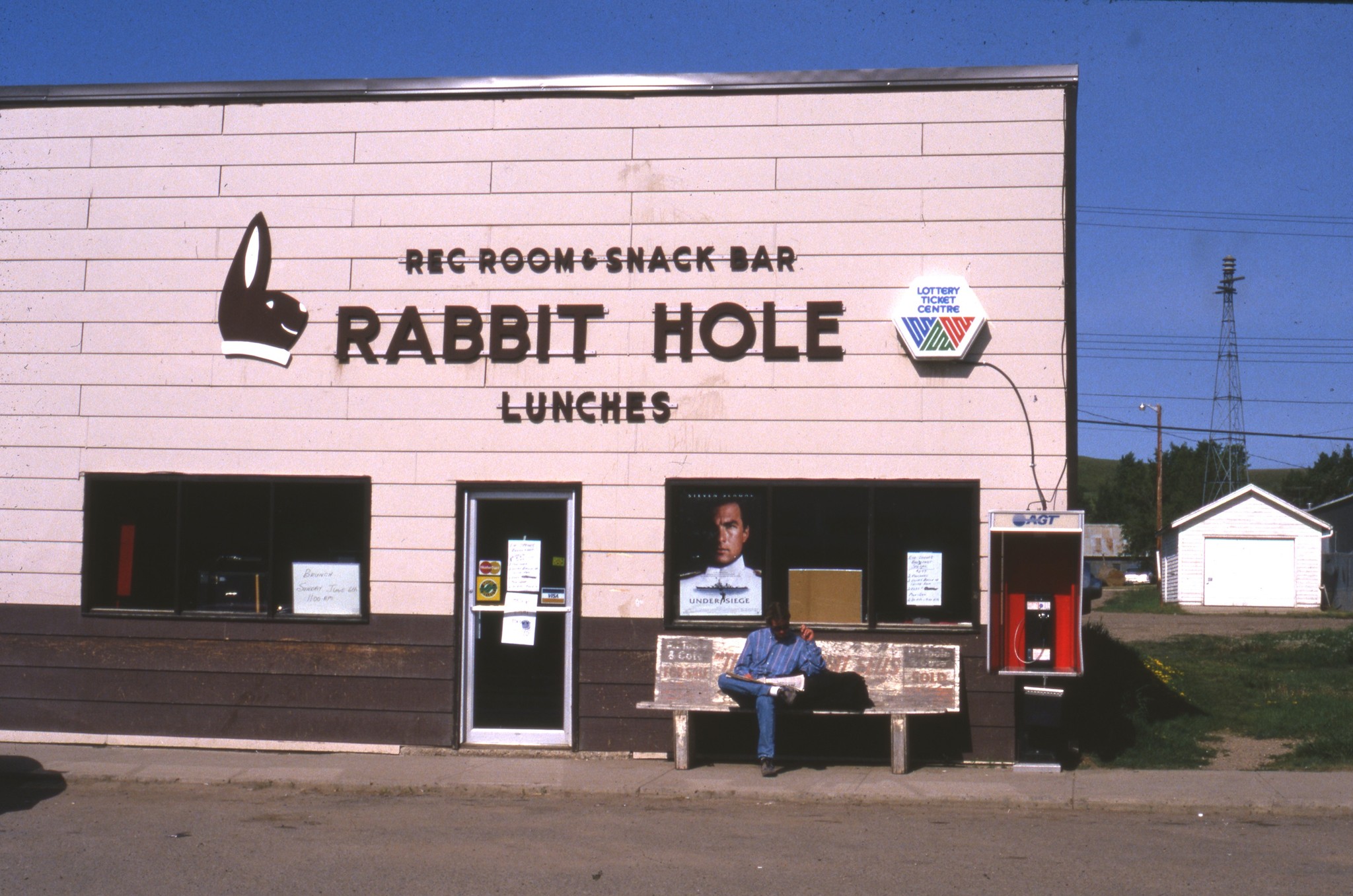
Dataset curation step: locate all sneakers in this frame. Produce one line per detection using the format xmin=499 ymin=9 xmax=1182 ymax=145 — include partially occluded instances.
xmin=776 ymin=687 xmax=794 ymax=705
xmin=760 ymin=758 xmax=774 ymax=777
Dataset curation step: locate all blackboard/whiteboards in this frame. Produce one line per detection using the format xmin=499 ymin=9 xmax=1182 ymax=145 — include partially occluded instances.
xmin=291 ymin=561 xmax=363 ymax=617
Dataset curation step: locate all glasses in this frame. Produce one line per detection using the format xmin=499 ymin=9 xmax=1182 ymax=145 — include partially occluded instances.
xmin=771 ymin=624 xmax=791 ymax=630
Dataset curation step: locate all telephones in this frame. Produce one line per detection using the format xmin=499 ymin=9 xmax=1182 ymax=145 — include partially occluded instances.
xmin=1040 ymin=612 xmax=1047 ymax=638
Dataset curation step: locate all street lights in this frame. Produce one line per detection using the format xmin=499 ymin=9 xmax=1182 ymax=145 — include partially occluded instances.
xmin=1139 ymin=402 xmax=1162 ymax=551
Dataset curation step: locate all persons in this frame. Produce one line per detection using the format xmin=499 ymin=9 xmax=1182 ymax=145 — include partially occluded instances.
xmin=674 ymin=494 xmax=762 ymax=616
xmin=718 ymin=601 xmax=825 ymax=776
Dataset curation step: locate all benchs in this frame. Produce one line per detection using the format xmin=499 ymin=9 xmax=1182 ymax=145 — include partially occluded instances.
xmin=638 ymin=632 xmax=963 ymax=775
